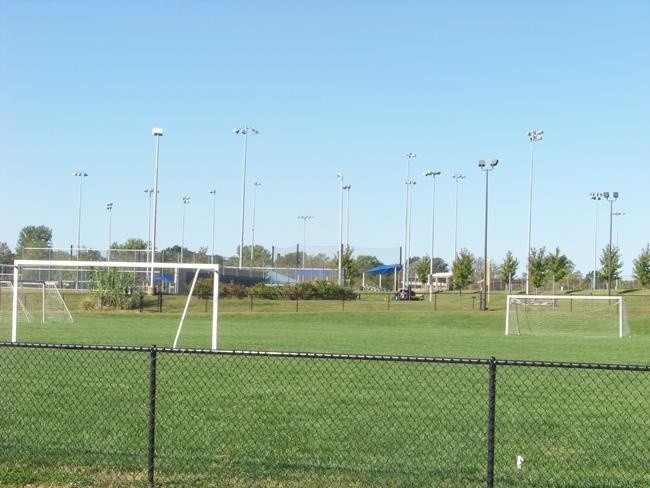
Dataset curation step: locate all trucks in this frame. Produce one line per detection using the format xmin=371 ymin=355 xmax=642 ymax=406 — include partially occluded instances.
xmin=392 ymin=289 xmax=424 ymax=301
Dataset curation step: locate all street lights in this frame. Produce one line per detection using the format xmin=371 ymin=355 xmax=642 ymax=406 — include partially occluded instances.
xmin=73 ymin=171 xmax=89 ymax=288
xmin=402 ymin=152 xmax=466 ymax=305
xmin=297 ymin=214 xmax=315 ymax=269
xmin=106 ymin=201 xmax=113 ymax=290
xmin=478 ymin=158 xmax=500 ymax=311
xmin=588 ymin=192 xmax=626 ymax=306
xmin=336 ymin=171 xmax=351 ymax=289
xmin=143 ymin=125 xmax=262 ymax=295
xmin=527 ymin=127 xmax=544 ymax=295
